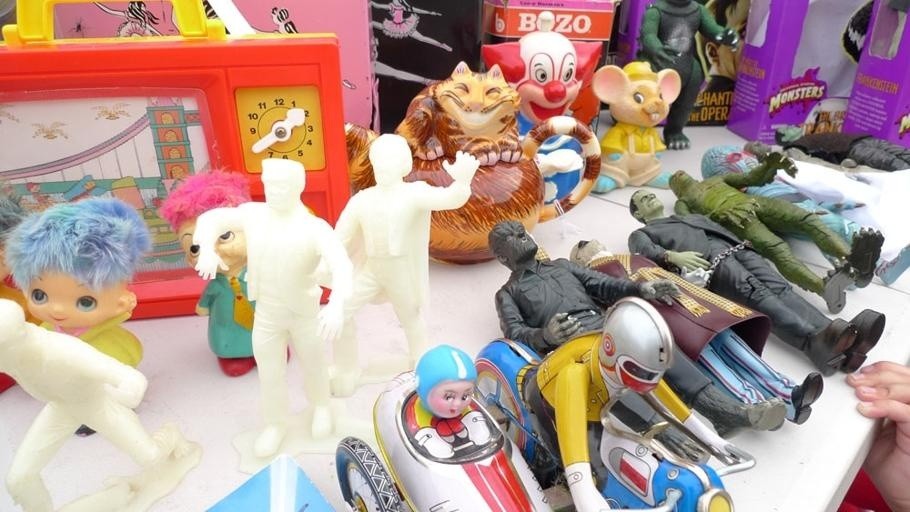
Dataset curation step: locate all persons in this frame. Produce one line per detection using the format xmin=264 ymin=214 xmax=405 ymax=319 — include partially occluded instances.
xmin=0 ymin=0 xmax=885 ymax=512
xmin=838 ymin=361 xmax=906 ymax=512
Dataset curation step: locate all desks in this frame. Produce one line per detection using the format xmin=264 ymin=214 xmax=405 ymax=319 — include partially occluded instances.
xmin=0 ymin=196 xmax=910 ymax=512
xmin=584 ymin=106 xmax=910 ymax=285
